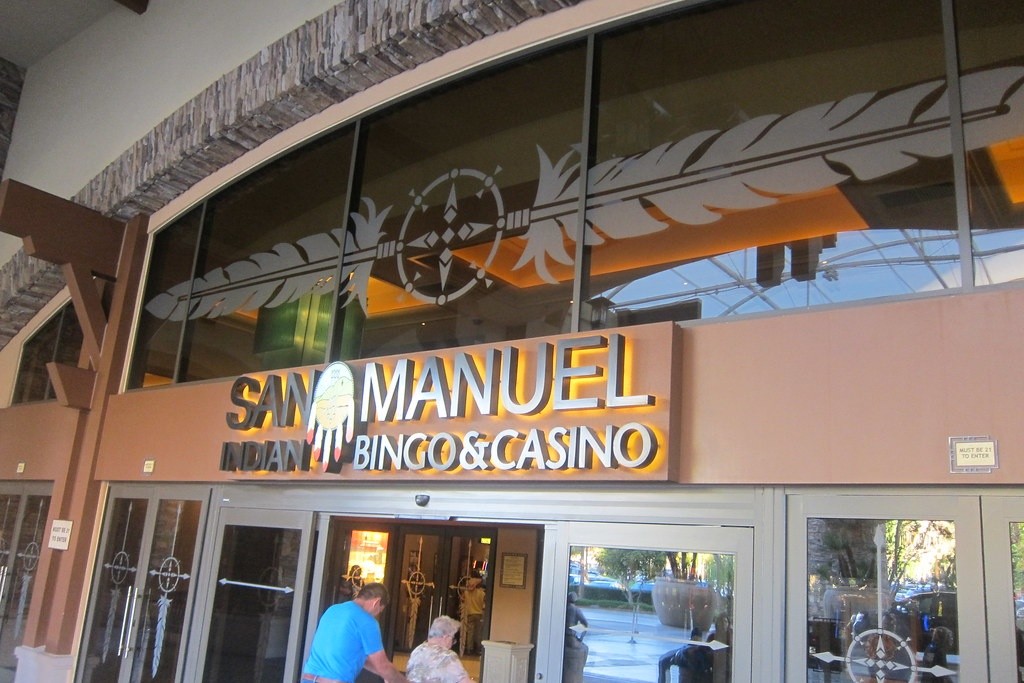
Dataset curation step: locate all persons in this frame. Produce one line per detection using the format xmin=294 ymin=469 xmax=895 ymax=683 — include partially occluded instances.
xmin=687 ymin=626 xmax=707 ymax=644
xmin=459 ymin=570 xmax=485 ymax=656
xmin=707 ymin=613 xmax=732 ymax=651
xmin=845 ymin=609 xmax=905 ymax=660
xmin=657 ymin=644 xmax=713 ymax=683
xmin=405 ymin=614 xmax=480 ymax=683
xmin=917 ymin=625 xmax=954 ymax=682
xmin=300 ymin=581 xmax=414 ymax=683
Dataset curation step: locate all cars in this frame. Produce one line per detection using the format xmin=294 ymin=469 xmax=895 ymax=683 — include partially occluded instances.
xmin=885 ymin=591 xmax=958 ymax=654
xmin=568 ymin=558 xmax=655 ymax=594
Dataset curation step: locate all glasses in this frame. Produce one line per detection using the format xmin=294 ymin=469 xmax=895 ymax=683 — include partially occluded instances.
xmin=449 ymin=635 xmax=457 ymax=645
